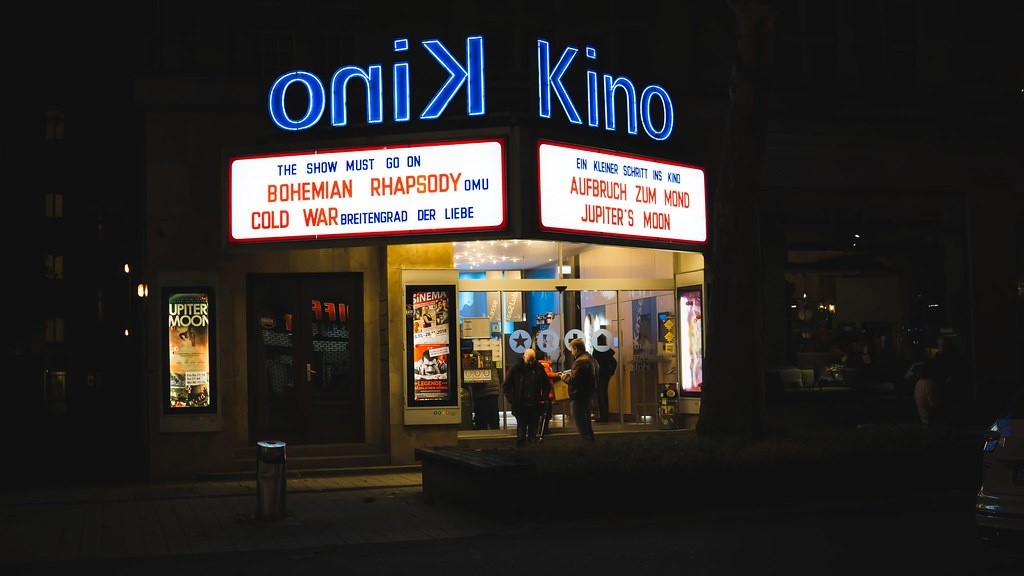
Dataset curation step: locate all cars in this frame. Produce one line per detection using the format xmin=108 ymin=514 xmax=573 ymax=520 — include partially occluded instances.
xmin=976 ymin=390 xmax=1024 ymax=544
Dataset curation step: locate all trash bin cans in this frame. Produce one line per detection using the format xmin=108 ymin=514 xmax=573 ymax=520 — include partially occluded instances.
xmin=255 ymin=440 xmax=287 ymax=519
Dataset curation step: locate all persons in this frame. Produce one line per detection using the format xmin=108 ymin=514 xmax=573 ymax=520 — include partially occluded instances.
xmin=414 ymin=350 xmax=447 ymax=380
xmin=592 ymin=345 xmax=617 ymax=423
xmin=471 ymin=369 xmax=501 ymax=429
xmin=460 ymin=325 xmax=474 ymax=388
xmin=558 ymin=338 xmax=597 ymax=457
xmin=913 ymin=336 xmax=961 ymax=424
xmin=502 ymin=349 xmax=550 ymax=452
xmin=535 ymin=351 xmax=563 ymax=444
xmin=423 ymin=298 xmax=448 ymax=328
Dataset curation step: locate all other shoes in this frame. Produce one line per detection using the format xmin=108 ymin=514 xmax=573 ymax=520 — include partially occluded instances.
xmin=594 ymin=418 xmax=608 ymax=423
xmin=537 ymin=431 xmax=541 ymax=435
xmin=544 ymin=430 xmax=552 ymax=435
